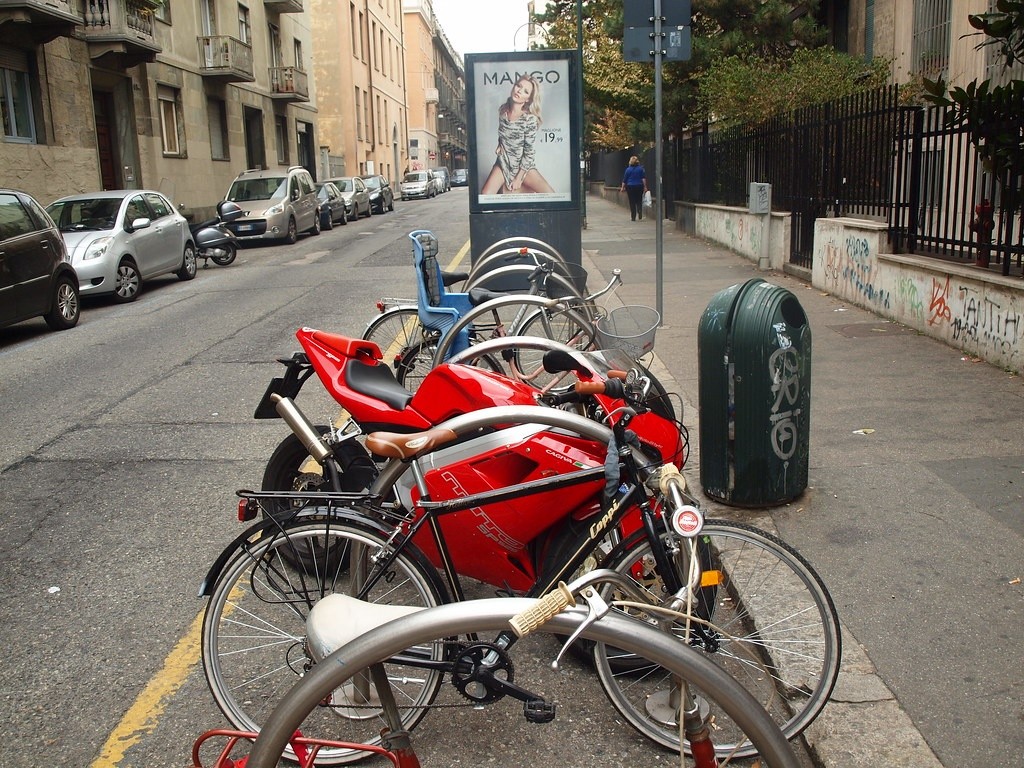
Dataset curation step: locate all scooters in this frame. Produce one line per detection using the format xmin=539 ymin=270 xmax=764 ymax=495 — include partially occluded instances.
xmin=171 ymin=199 xmax=244 ymax=268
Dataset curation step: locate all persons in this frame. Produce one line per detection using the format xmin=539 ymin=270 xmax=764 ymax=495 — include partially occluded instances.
xmin=620 ymin=156 xmax=648 ymax=221
xmin=480 ymin=75 xmax=556 ymax=194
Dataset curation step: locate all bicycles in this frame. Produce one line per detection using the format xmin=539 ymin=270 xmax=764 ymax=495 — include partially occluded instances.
xmin=195 ymin=228 xmax=845 ymax=768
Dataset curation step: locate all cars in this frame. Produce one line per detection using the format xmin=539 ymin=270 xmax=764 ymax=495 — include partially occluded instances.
xmin=0 ymin=188 xmax=80 ymax=334
xmin=448 ymin=168 xmax=469 ymax=186
xmin=313 ymin=182 xmax=347 ymax=230
xmin=399 ymin=167 xmax=450 ymax=199
xmin=325 ymin=176 xmax=373 ymax=222
xmin=41 ymin=189 xmax=197 ymax=304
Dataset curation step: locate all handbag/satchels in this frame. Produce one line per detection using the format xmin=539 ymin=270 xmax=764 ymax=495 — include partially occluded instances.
xmin=641 ymin=190 xmax=652 ymax=209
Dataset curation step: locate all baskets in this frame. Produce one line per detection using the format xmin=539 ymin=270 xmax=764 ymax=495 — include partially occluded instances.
xmin=595 ymin=304 xmax=661 ymax=362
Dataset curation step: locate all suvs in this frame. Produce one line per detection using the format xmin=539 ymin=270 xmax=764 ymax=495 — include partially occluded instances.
xmin=220 ymin=165 xmax=321 ymax=244
xmin=357 ymin=173 xmax=395 ymax=214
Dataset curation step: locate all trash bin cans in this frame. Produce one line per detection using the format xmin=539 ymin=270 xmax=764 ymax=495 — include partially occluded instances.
xmin=698 ymin=278 xmax=812 ymax=509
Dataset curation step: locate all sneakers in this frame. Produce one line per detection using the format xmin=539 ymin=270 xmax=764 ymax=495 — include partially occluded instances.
xmin=638 ymin=210 xmax=642 ymax=220
xmin=631 ymin=216 xmax=636 ymax=221
